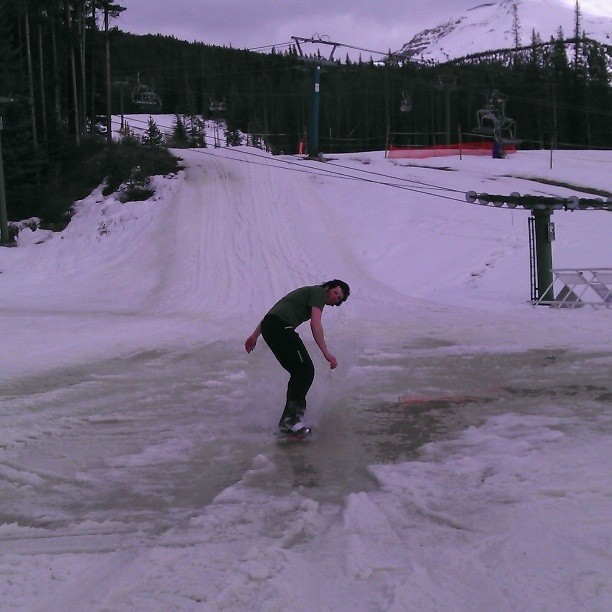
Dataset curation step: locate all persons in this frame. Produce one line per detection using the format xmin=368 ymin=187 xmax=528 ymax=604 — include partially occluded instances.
xmin=244 ymin=278 xmax=350 ymax=436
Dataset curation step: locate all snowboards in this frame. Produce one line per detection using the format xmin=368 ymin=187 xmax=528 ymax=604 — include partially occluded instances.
xmin=270 ymin=416 xmax=314 ymax=443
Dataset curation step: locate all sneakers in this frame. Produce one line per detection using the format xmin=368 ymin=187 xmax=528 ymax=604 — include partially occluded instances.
xmin=279 ymin=399 xmax=311 ymax=438
